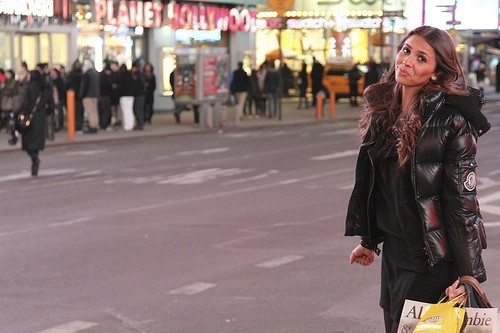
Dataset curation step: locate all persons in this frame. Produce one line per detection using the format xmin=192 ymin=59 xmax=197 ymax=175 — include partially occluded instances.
xmin=232 ymin=58 xmax=283 ymax=124
xmin=68 ymin=57 xmax=156 ymax=133
xmin=9 ymin=70 xmax=54 ymax=175
xmin=344 ymin=25 xmax=492 ymax=333
xmin=297 ymin=62 xmax=310 ymax=108
xmin=348 ymin=62 xmax=361 ymax=106
xmin=0 ymin=61 xmax=71 ymax=145
xmin=169 ymin=66 xmax=191 ymax=123
xmin=311 ymin=56 xmax=324 ymax=106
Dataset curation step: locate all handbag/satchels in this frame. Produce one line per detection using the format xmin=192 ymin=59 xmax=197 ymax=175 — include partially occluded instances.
xmin=16 ymin=111 xmax=31 ymax=135
xmin=413 ymin=292 xmax=467 ymax=333
xmin=397 ymin=293 xmax=499 ymax=333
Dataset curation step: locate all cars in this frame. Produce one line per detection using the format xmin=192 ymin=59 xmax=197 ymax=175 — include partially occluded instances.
xmin=315 ymin=64 xmax=365 ymax=102
